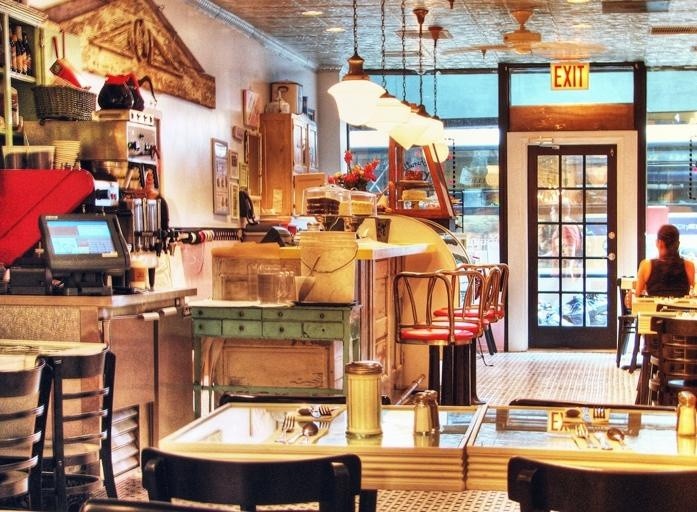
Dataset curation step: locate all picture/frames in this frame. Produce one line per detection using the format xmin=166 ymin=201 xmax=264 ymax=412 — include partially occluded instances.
xmin=205 ymin=90 xmax=263 ymax=221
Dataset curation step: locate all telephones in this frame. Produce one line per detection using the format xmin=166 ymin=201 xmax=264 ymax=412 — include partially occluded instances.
xmin=239 ymin=190 xmax=253 ymax=217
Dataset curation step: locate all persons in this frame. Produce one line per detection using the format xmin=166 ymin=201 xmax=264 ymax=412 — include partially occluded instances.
xmin=550 ymin=198 xmax=584 ymax=281
xmin=635 ymin=224 xmax=696 ymax=298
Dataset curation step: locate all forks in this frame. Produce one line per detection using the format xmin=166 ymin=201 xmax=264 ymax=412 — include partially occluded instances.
xmin=317 ymin=405 xmax=336 ymax=416
xmin=274 ymin=415 xmax=295 ymax=444
xmin=312 ymin=419 xmax=331 ymax=446
xmin=576 ymin=406 xmax=612 ymax=452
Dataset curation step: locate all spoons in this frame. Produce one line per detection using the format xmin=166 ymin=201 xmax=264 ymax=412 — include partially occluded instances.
xmin=607 ymin=427 xmax=632 ymax=452
xmin=298 ymin=406 xmax=314 ymax=416
xmin=303 ymin=420 xmax=318 ymax=447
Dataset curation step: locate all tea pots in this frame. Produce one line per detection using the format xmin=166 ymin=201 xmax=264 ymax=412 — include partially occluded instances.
xmin=125 ymin=71 xmax=157 ymax=110
xmin=98 ymin=75 xmax=135 ymax=109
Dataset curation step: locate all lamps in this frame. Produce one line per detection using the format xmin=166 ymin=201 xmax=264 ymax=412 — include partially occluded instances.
xmin=326 ymin=1 xmax=449 ymax=164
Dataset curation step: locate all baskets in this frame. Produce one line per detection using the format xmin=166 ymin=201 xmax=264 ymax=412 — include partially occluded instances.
xmin=31 ymin=85 xmax=97 ymax=121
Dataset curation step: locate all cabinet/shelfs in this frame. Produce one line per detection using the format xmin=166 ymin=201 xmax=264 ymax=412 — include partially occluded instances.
xmin=186 ymin=297 xmax=361 ymax=419
xmin=386 ymin=137 xmax=457 ymax=219
xmin=260 ymin=111 xmax=319 ymax=221
xmin=1 ymin=1 xmax=51 ymax=157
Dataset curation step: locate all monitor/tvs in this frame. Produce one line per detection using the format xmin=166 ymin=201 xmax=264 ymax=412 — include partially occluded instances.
xmin=38 ymin=213 xmax=130 ymax=295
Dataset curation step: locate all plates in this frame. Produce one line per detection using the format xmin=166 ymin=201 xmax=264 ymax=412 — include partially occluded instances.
xmin=52 ymin=140 xmax=80 ymax=169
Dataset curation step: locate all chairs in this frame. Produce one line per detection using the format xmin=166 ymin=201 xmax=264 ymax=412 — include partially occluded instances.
xmin=395 ymin=259 xmax=509 ymax=407
xmin=618 ymin=278 xmax=697 ymax=403
xmin=506 ymin=456 xmax=697 ymax=512
xmin=139 ymin=448 xmax=377 ymax=512
xmin=0 ymin=359 xmax=55 ymax=511
xmin=42 ymin=353 xmax=121 ymax=512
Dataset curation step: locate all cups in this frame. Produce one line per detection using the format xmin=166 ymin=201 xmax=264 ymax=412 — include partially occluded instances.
xmin=308 ymin=222 xmax=322 ymax=231
xmin=295 ymin=276 xmax=317 ymax=307
xmin=375 ymin=216 xmax=391 ymax=243
xmin=256 ymin=261 xmax=295 ymax=305
xmin=626 ymin=412 xmax=641 ymax=436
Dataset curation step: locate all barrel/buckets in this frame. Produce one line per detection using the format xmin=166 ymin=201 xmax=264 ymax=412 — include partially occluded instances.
xmin=298 ymin=231 xmax=358 ymax=305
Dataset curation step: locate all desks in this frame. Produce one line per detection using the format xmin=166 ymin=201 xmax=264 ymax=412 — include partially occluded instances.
xmin=155 ymin=402 xmax=481 ymax=495
xmin=465 ymin=406 xmax=697 ymax=491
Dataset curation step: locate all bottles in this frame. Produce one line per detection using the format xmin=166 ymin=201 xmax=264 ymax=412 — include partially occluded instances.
xmin=675 ymin=391 xmax=692 ymax=432
xmin=344 ymin=360 xmax=383 ymax=440
xmin=347 ymin=437 xmax=381 ymax=452
xmin=424 ymin=389 xmax=439 ymax=429
xmin=6 ymin=23 xmax=32 ymax=76
xmin=429 ymin=431 xmax=439 ymax=448
xmin=414 ymin=392 xmax=432 ymax=435
xmin=677 ymin=394 xmax=697 ymax=438
xmin=416 ymin=435 xmax=432 ymax=449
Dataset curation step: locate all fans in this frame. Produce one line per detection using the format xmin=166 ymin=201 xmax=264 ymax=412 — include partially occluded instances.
xmin=439 ymin=10 xmax=605 ymax=60
